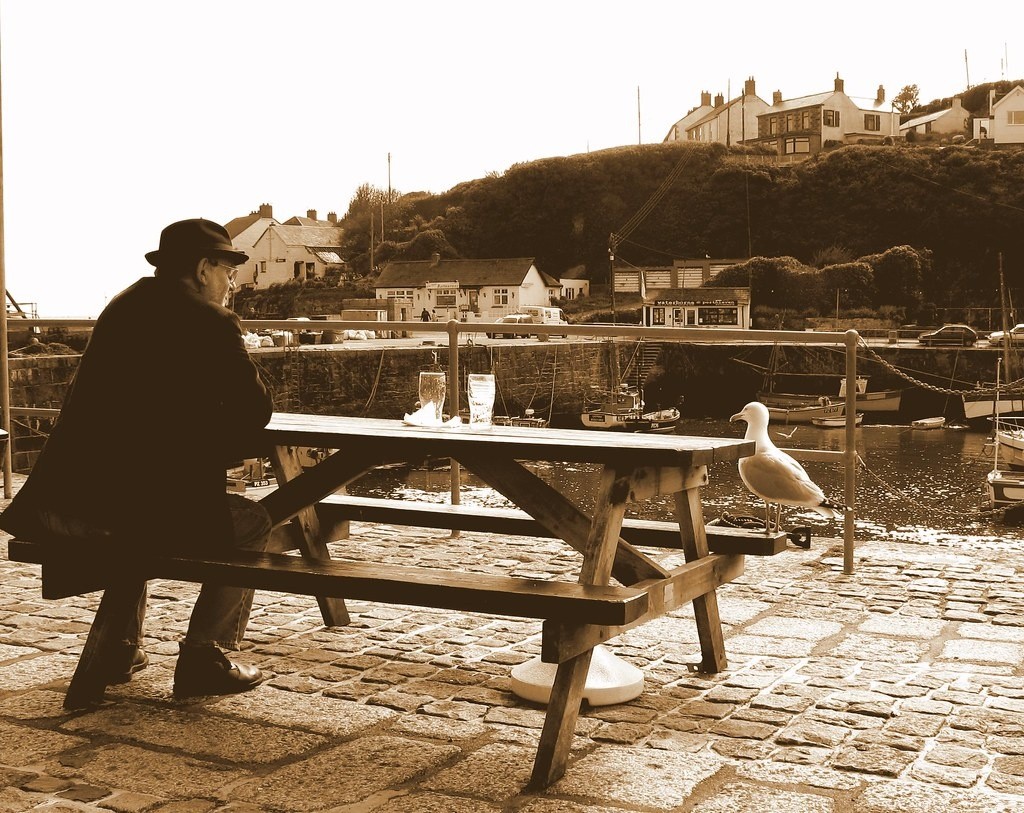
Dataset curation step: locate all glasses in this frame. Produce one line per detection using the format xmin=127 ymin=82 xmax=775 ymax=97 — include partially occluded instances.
xmin=207 ymin=259 xmax=239 ymax=283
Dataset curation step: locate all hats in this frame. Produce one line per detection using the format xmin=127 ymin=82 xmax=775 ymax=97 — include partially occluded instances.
xmin=145 ymin=219 xmax=249 ymax=268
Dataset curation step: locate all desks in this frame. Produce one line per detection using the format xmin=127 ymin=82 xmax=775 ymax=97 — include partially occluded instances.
xmin=62 ymin=412 xmax=758 ymax=790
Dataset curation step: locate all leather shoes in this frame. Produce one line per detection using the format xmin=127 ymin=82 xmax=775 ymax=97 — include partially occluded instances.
xmin=107 ymin=647 xmax=149 ymax=683
xmin=172 ymin=641 xmax=263 ymax=697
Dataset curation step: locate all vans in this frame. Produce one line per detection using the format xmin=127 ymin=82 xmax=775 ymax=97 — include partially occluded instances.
xmin=519 ymin=305 xmax=568 ymax=343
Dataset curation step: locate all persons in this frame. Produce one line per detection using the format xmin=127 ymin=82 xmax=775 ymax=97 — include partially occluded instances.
xmin=0 ymin=219 xmax=275 ymax=700
xmin=420 ymin=308 xmax=431 ymax=322
xmin=431 ymin=309 xmax=438 ymax=322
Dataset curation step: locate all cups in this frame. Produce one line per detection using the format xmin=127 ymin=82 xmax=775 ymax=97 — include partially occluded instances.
xmin=467 ymin=374 xmax=495 ymax=427
xmin=419 ymin=373 xmax=447 ymax=423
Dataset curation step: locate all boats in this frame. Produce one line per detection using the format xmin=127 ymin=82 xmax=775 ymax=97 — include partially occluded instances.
xmin=912 ymin=416 xmax=946 ymax=429
xmin=580 ymin=384 xmax=681 ymax=435
xmin=811 ymin=412 xmax=864 ymax=428
xmin=229 ymin=368 xmax=551 ymax=501
xmin=738 ymin=253 xmax=1024 ymax=510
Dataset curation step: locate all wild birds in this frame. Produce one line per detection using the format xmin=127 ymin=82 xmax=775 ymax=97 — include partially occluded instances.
xmin=730 ymin=401 xmax=854 ymax=536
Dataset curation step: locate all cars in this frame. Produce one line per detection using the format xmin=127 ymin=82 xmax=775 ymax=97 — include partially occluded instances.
xmin=486 ymin=314 xmax=533 ymax=340
xmin=918 ymin=323 xmax=978 ymax=347
xmin=988 ymin=323 xmax=1024 ymax=347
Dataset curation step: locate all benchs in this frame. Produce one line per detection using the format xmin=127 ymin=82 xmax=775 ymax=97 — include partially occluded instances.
xmin=8 ymin=538 xmax=650 ymax=627
xmin=317 ymin=495 xmax=790 ymax=557
